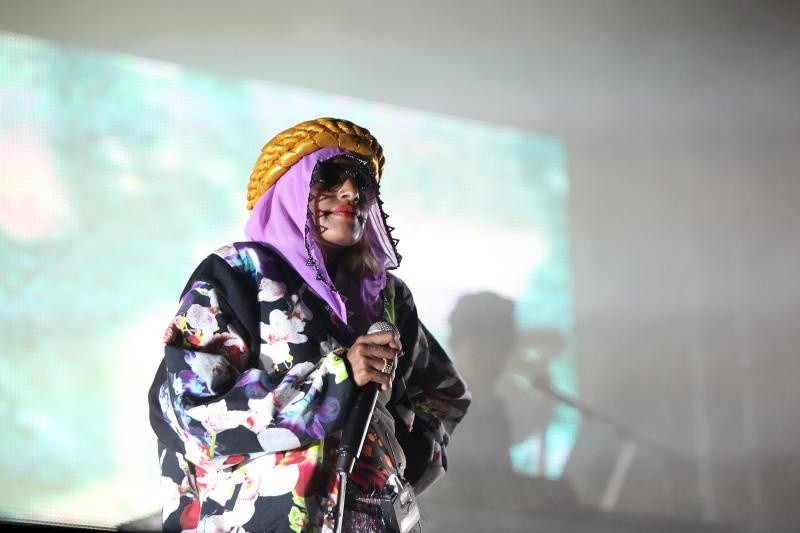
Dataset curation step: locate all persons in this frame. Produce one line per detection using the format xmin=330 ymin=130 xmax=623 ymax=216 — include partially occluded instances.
xmin=149 ymin=117 xmax=471 ymax=533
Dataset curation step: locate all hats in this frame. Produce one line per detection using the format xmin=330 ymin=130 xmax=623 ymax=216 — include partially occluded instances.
xmin=247 ymin=117 xmax=385 ymax=215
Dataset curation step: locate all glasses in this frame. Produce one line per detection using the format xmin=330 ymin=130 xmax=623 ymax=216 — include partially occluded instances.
xmin=312 ymin=162 xmax=371 ymax=192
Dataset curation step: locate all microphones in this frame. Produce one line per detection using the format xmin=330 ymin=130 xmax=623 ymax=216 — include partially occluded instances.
xmin=333 ymin=320 xmax=403 ymax=476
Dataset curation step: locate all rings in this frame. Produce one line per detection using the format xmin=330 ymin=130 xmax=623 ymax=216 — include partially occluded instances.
xmin=382 ymin=358 xmax=393 ymax=374
